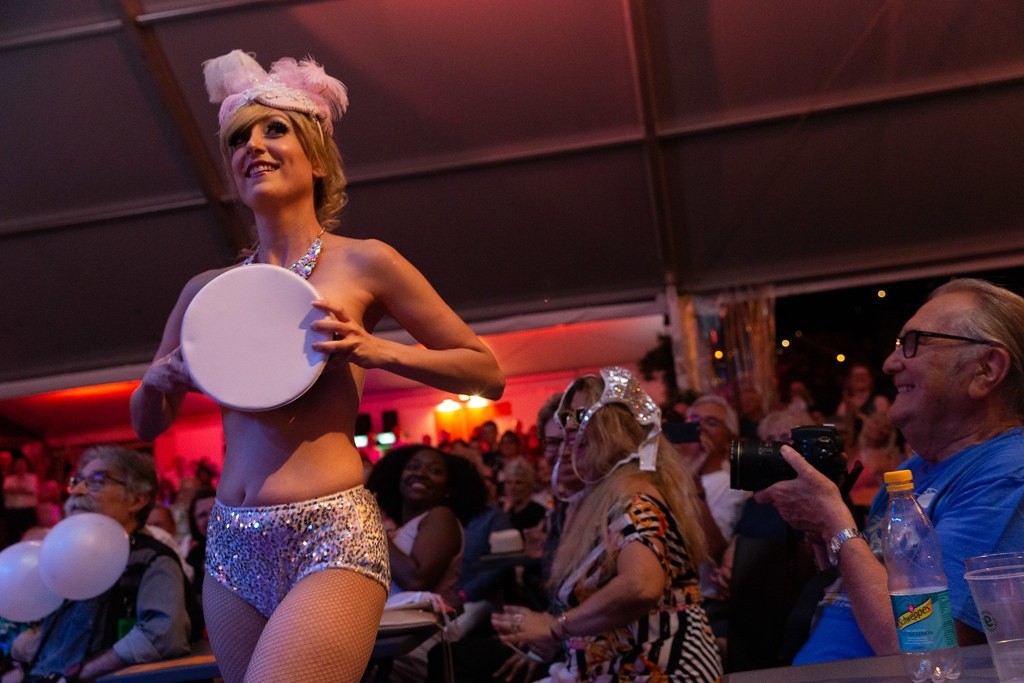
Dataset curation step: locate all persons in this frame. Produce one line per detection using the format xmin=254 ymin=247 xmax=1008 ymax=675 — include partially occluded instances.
xmin=0 ymin=443 xmax=191 ymax=683
xmin=789 ymin=279 xmax=1023 ymax=661
xmin=130 ymin=49 xmax=505 ymax=683
xmin=493 ymin=370 xmax=728 ymax=683
xmin=361 ymin=445 xmax=466 ymax=617
xmin=1 ymin=250 xmax=1024 ymax=578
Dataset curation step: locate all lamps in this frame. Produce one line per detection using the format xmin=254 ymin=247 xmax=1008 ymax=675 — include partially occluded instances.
xmin=376 ymin=410 xmax=401 ymax=445
xmin=353 ymin=413 xmax=371 ymax=447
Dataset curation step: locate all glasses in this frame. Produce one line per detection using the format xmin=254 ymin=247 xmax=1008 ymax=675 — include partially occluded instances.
xmin=558 ymin=406 xmax=590 ymax=427
xmin=70 ymin=472 xmax=132 ymax=492
xmin=894 ymin=329 xmax=1016 ymax=364
xmin=686 ymin=415 xmax=732 ymax=438
xmin=540 ymin=436 xmax=577 ymax=451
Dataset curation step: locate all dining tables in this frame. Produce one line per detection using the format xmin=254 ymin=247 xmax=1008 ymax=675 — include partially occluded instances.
xmin=715 ymin=641 xmax=1022 ymax=683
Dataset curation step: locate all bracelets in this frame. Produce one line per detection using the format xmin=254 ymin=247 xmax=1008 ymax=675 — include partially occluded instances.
xmin=549 ymin=611 xmax=572 ymax=641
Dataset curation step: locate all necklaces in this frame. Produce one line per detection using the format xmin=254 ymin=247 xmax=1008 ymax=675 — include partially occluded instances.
xmin=240 ymin=223 xmax=327 ymax=280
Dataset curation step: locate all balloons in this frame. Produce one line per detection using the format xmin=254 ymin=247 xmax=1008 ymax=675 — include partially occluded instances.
xmin=0 ymin=542 xmax=64 ymax=624
xmin=36 ymin=513 xmax=131 ymax=601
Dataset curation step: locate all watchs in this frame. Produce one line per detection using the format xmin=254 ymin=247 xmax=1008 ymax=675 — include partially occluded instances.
xmin=828 ymin=527 xmax=870 ymax=566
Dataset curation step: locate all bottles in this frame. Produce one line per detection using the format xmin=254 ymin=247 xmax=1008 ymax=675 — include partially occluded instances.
xmin=885 ymin=469 xmax=960 ymax=683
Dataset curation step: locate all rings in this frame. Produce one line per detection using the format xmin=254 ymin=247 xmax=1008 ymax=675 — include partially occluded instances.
xmin=512 ymin=614 xmax=524 ymax=631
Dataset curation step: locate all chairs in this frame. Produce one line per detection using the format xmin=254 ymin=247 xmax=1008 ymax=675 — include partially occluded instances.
xmin=92 ymin=545 xmax=544 ymax=683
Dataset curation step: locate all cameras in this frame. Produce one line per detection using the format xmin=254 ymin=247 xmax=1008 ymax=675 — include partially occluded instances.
xmin=730 ymin=423 xmax=844 ymax=497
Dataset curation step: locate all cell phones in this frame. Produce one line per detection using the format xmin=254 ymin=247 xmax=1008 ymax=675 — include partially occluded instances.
xmin=662 ymin=422 xmax=701 ymax=443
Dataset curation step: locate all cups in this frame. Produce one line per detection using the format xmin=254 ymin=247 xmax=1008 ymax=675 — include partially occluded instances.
xmin=962 ymin=550 xmax=1024 ymax=683
